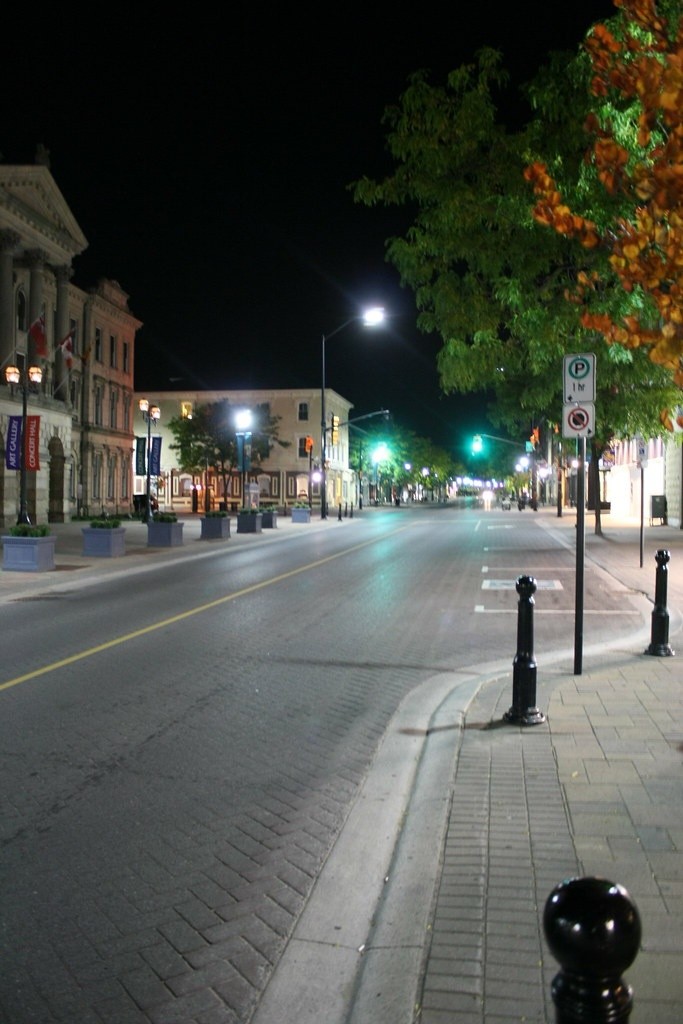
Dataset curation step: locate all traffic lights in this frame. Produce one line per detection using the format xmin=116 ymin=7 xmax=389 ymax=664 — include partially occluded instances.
xmin=473 ymin=436 xmax=482 ymax=454
xmin=330 ymin=414 xmax=339 ymax=445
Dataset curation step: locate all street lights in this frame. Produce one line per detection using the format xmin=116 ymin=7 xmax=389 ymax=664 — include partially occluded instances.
xmin=138 ymin=398 xmax=161 ymax=524
xmin=320 ymin=308 xmax=385 ymax=520
xmin=235 ymin=415 xmax=253 ymax=507
xmin=7 ymin=352 xmax=43 ymax=528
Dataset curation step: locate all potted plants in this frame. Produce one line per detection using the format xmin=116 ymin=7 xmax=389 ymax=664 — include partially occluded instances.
xmin=291 ymin=504 xmax=311 ymax=523
xmin=200 ymin=512 xmax=231 ymax=538
xmin=236 ymin=507 xmax=278 ymax=533
xmin=81 ymin=519 xmax=127 ymax=559
xmin=1 ymin=524 xmax=58 ymax=572
xmin=146 ymin=512 xmax=185 ymax=547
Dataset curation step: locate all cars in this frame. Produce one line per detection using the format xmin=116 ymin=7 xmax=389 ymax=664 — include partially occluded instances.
xmin=131 ymin=494 xmax=159 ymax=514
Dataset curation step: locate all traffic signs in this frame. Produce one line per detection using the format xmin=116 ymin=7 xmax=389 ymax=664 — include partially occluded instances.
xmin=564 ymin=352 xmax=596 ymax=403
